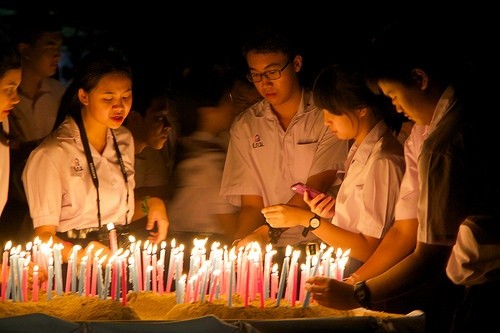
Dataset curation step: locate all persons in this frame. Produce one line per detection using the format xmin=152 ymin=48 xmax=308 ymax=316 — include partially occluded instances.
xmin=1 ymin=11 xmax=500 ymax=332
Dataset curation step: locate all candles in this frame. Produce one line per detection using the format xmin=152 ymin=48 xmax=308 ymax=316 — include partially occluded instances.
xmin=1 ymin=221 xmax=352 ymax=308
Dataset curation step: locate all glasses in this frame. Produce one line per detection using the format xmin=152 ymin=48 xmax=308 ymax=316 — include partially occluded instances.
xmin=245 ymin=56 xmax=292 ymax=83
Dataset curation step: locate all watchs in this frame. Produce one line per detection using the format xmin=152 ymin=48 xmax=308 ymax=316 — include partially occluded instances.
xmin=353 ymin=280 xmax=373 ymax=310
xmin=301 ymin=212 xmax=321 ymax=237
